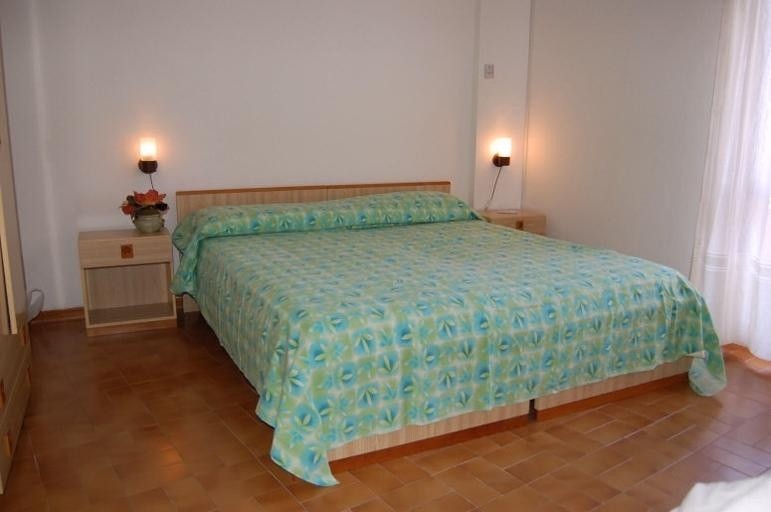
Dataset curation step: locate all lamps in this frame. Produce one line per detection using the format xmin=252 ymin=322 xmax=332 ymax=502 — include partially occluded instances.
xmin=491 ymin=135 xmax=514 ymax=169
xmin=135 ymin=134 xmax=159 ymax=176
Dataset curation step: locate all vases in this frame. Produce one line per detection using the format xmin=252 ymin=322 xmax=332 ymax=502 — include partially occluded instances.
xmin=129 ymin=210 xmax=166 ymax=234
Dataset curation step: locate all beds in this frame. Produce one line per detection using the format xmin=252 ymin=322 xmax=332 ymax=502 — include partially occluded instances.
xmin=170 ymin=177 xmax=728 ymax=490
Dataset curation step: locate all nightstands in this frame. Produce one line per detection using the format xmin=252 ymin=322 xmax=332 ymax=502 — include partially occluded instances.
xmin=76 ymin=229 xmax=180 ymax=339
xmin=473 ymin=206 xmax=549 ymax=239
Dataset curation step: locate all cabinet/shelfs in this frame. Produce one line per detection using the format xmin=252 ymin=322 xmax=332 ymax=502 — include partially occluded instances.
xmin=0 ymin=19 xmax=40 ymax=500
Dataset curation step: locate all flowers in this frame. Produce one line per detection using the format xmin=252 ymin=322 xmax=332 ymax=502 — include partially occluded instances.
xmin=120 ymin=186 xmax=171 ymax=222
